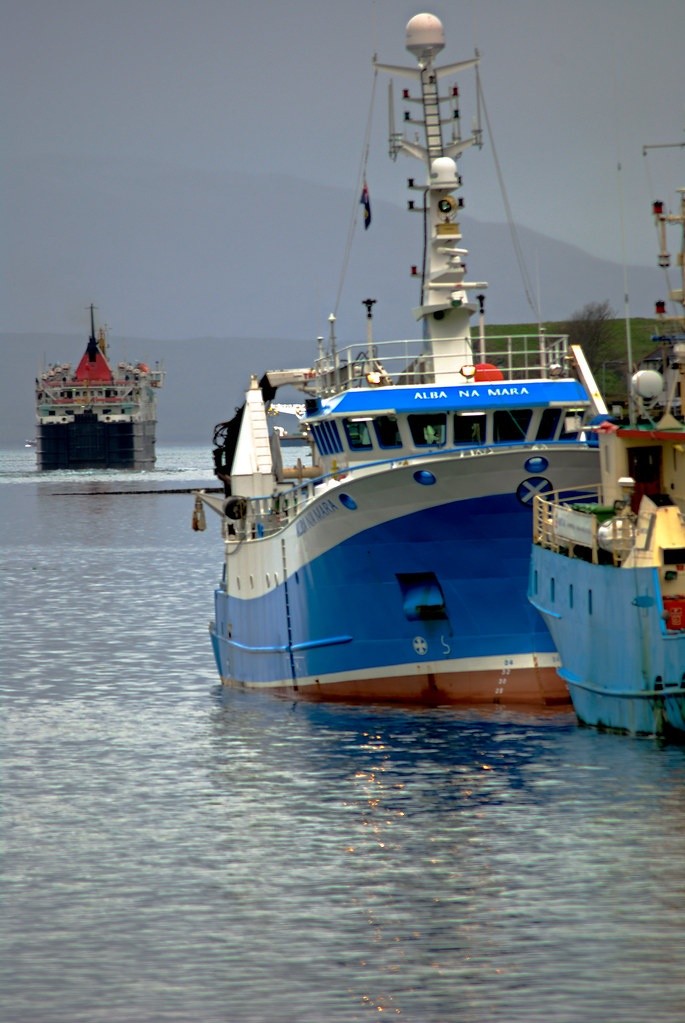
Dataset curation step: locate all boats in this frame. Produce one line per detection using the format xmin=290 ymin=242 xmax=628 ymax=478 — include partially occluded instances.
xmin=189 ymin=10 xmax=628 ymax=708
xmin=530 ymin=136 xmax=685 ymax=737
xmin=33 ymin=303 xmax=166 ymax=470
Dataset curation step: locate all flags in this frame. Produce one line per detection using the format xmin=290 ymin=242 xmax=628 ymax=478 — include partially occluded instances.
xmin=360 ymin=183 xmax=372 ymax=230
